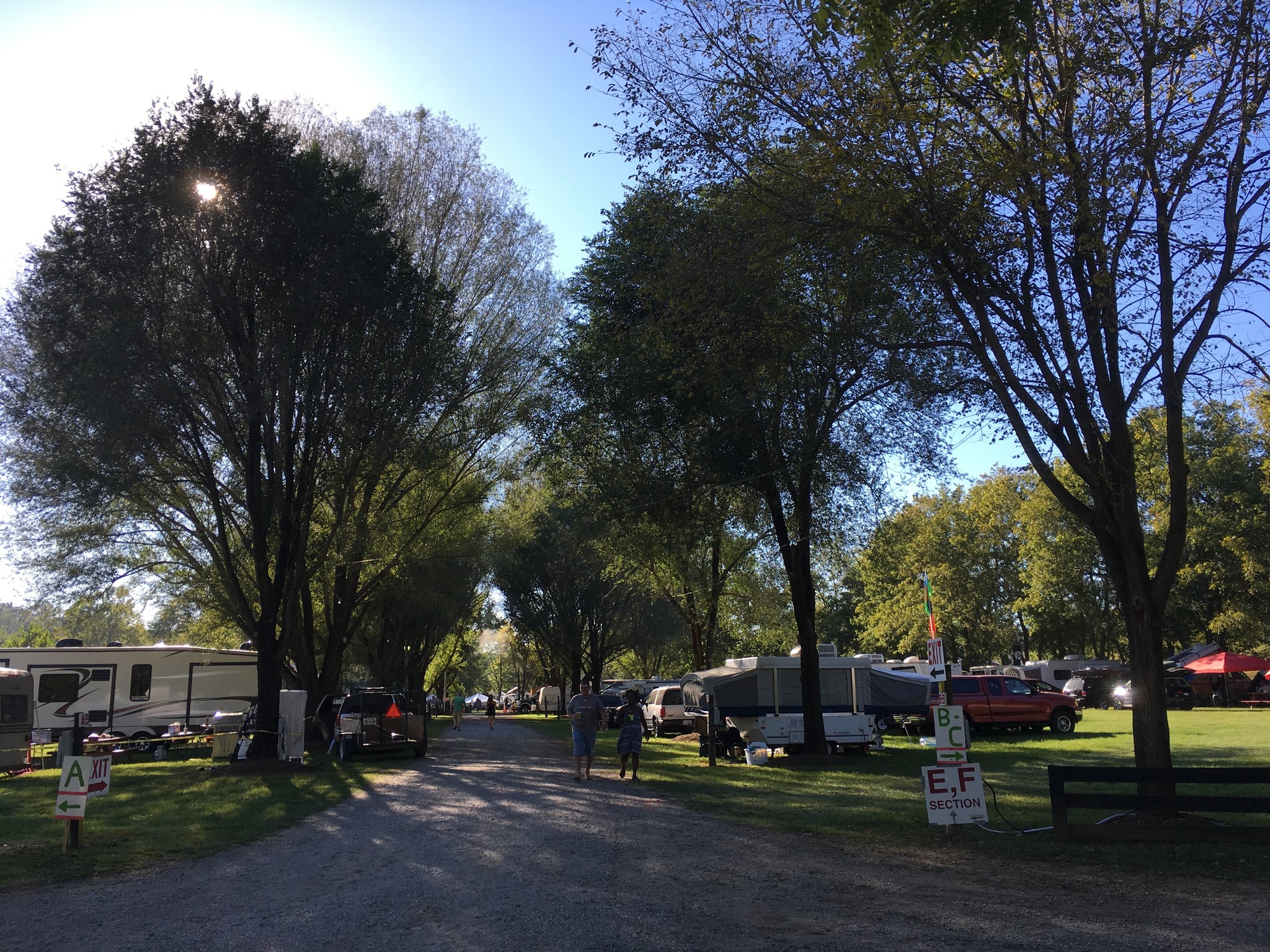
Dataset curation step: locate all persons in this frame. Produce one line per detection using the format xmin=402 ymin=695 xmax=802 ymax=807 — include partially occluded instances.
xmin=566 ymin=680 xmax=607 ymax=781
xmin=446 ymin=690 xmax=517 ymax=731
xmin=1211 ymin=673 xmax=1270 ymax=707
xmin=615 ymin=689 xmax=650 ymax=780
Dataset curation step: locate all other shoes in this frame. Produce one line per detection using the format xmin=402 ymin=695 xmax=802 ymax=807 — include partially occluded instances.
xmin=620 ymin=764 xmax=627 ymax=778
xmin=574 ymin=774 xmax=581 ymax=780
xmin=491 ymin=726 xmax=494 ymax=730
xmin=585 ymin=773 xmax=592 ymax=780
xmin=631 ymin=776 xmax=639 ymax=780
xmin=457 ymin=729 xmax=462 ymax=731
xmin=452 ymin=726 xmax=457 ymax=730
xmin=489 ymin=729 xmax=492 ymax=731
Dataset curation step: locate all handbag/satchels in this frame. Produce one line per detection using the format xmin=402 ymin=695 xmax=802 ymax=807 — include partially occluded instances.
xmin=485 ymin=711 xmax=489 ymax=716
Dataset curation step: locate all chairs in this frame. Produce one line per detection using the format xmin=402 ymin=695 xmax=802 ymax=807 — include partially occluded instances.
xmin=190 ymin=728 xmax=209 ymax=756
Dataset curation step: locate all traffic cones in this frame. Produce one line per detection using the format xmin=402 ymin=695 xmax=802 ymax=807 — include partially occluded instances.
xmin=504 ymin=705 xmax=507 ymax=713
xmin=463 ymin=707 xmax=465 ymax=713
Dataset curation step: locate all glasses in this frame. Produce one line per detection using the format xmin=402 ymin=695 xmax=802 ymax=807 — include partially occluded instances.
xmin=580 ymin=686 xmax=589 ymax=689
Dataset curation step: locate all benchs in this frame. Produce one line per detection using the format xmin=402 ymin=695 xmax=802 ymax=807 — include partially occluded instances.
xmin=87 ymin=747 xmax=139 ymax=757
xmin=1241 ymin=700 xmax=1270 ymax=704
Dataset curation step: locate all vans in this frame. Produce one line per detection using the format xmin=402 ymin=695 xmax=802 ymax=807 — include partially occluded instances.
xmin=0 ymin=668 xmax=34 ymax=772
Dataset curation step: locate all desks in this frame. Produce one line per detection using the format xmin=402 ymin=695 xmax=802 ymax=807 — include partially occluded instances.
xmin=83 ymin=736 xmax=132 ymax=761
xmin=161 ymin=731 xmax=193 ymax=758
xmin=30 ymin=742 xmax=55 ymax=770
xmin=1247 ymin=692 xmax=1270 ymax=709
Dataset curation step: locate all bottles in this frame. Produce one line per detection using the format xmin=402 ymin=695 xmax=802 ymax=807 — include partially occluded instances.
xmin=183 ymin=723 xmax=187 ymax=733
xmin=206 ymin=717 xmax=208 ymax=726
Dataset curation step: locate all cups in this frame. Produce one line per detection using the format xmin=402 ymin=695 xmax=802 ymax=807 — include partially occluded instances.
xmin=575 ymin=712 xmax=581 ymax=719
xmin=628 ymin=714 xmax=634 ymax=721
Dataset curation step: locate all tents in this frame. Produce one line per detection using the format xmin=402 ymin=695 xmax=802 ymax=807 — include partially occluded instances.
xmin=1183 ymin=652 xmax=1270 ymax=708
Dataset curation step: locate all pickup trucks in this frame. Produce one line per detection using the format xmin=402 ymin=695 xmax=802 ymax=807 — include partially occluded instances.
xmin=334 ymin=687 xmax=429 ymax=758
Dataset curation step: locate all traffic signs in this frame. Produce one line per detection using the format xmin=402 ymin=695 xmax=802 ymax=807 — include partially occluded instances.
xmin=55 ymin=756 xmax=93 ymax=820
xmin=88 ymin=755 xmax=112 ymax=796
xmin=931 ymin=706 xmax=968 ymax=765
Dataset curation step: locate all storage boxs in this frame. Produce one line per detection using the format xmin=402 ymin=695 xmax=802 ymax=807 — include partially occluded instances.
xmin=169 ymin=724 xmax=180 ymax=734
xmin=744 ymin=747 xmax=768 ymax=765
xmin=32 ymin=730 xmax=52 ymax=743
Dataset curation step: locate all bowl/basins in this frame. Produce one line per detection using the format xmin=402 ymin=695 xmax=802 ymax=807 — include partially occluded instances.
xmin=88 ymin=737 xmax=99 ymax=741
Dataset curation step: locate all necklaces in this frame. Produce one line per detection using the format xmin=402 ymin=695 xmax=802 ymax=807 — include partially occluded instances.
xmin=627 ymin=705 xmax=634 ymax=712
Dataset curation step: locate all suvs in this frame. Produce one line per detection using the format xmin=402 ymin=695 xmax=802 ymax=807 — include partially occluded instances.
xmin=614 ymin=684 xmax=695 ymax=738
xmin=592 ymin=692 xmax=625 ymax=726
xmin=1059 ymin=665 xmax=1198 ymax=712
xmin=516 ymin=692 xmax=540 ymax=714
xmin=925 ymin=675 xmax=1083 ymax=734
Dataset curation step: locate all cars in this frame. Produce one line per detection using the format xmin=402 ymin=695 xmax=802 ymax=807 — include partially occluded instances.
xmin=1005 ymin=679 xmax=1063 ymax=695
xmin=309 ymin=693 xmax=350 ymax=742
